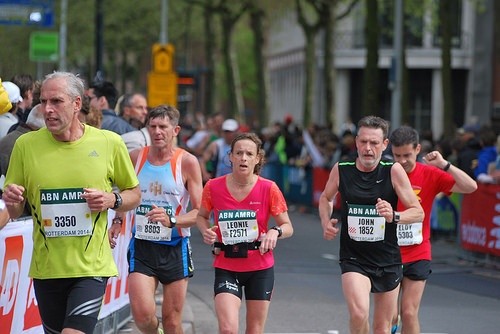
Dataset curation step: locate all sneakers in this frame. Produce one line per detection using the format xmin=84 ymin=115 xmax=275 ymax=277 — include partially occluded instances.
xmin=390 ymin=315 xmax=401 ymax=334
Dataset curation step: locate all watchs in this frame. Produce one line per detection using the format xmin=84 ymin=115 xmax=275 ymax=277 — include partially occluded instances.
xmin=392 ymin=211 xmax=400 ymax=224
xmin=270 ymin=225 xmax=282 ymax=237
xmin=110 ymin=191 xmax=123 ymax=210
xmin=168 ymin=215 xmax=177 ymax=228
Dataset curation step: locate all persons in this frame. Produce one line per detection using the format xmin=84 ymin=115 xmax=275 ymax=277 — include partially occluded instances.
xmin=107 ymin=104 xmax=205 ymax=334
xmin=3 ymin=71 xmax=142 ymax=333
xmin=318 ymin=116 xmax=426 ymax=334
xmin=77 ymin=103 xmax=104 ymax=129
xmin=417 ymin=127 xmax=500 ymax=182
xmin=110 ymin=107 xmax=151 ymax=193
xmin=199 ymin=118 xmax=243 ymax=188
xmin=390 ymin=125 xmax=478 ymax=334
xmin=196 ymin=131 xmax=295 ymax=334
xmin=0 ymin=77 xmax=24 ymax=139
xmin=179 ymin=110 xmax=358 ymax=214
xmin=119 ymin=91 xmax=149 ymax=131
xmin=0 ymin=103 xmax=47 ymax=217
xmin=88 ymin=80 xmax=139 ymax=135
xmin=1 ymin=78 xmax=13 ymax=115
xmin=11 ymin=73 xmax=35 ymax=123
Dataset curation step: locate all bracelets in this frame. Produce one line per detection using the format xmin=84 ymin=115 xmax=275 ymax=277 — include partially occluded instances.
xmin=443 ymin=162 xmax=451 ymax=171
xmin=112 ymin=217 xmax=123 ymax=224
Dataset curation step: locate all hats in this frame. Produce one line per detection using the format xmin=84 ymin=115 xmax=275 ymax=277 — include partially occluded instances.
xmin=223 ymin=119 xmax=238 ymax=132
xmin=2 ymin=81 xmax=23 ymax=104
